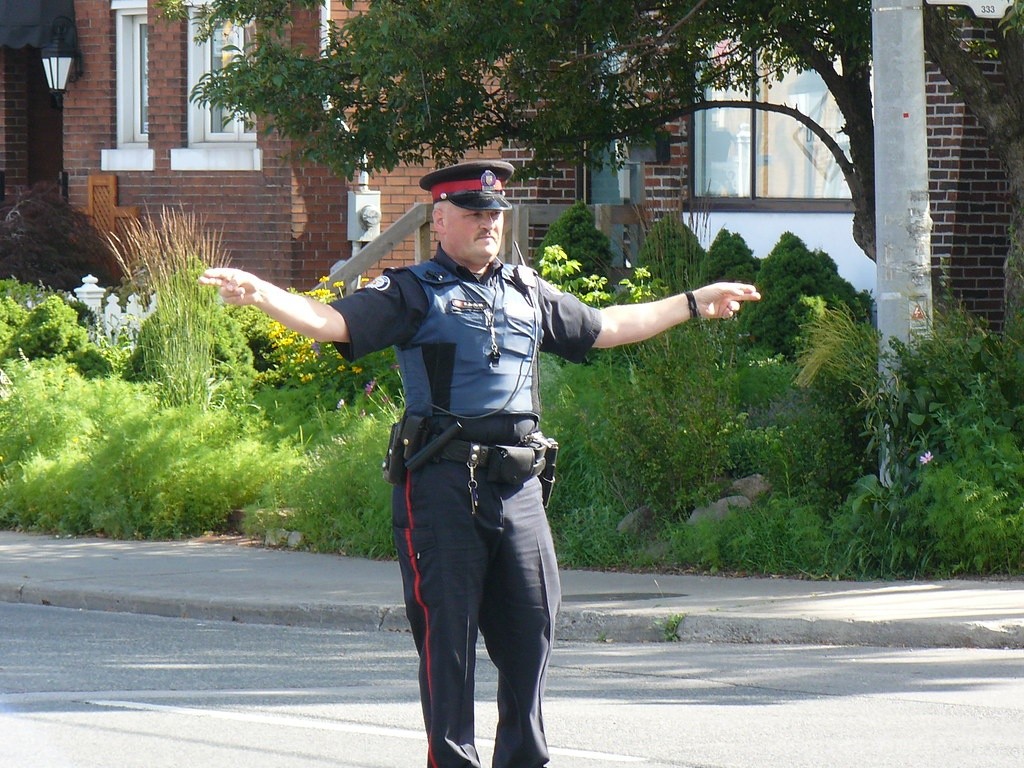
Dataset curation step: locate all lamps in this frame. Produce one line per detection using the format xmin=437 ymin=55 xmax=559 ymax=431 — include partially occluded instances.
xmin=41 ymin=16 xmax=83 ymax=103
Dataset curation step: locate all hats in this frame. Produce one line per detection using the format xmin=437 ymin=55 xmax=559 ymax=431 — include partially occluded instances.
xmin=418 ymin=160 xmax=514 ymax=211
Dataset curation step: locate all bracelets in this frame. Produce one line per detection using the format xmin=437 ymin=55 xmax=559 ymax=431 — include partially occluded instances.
xmin=684 ymin=291 xmax=701 ymax=320
xmin=197 ymin=161 xmax=760 ymax=768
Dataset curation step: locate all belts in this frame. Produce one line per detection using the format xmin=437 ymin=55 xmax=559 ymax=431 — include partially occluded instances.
xmin=425 ymin=434 xmax=547 ymax=468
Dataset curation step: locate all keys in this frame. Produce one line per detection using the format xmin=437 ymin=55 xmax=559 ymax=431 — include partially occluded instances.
xmin=467 ymin=480 xmax=479 ymax=513
